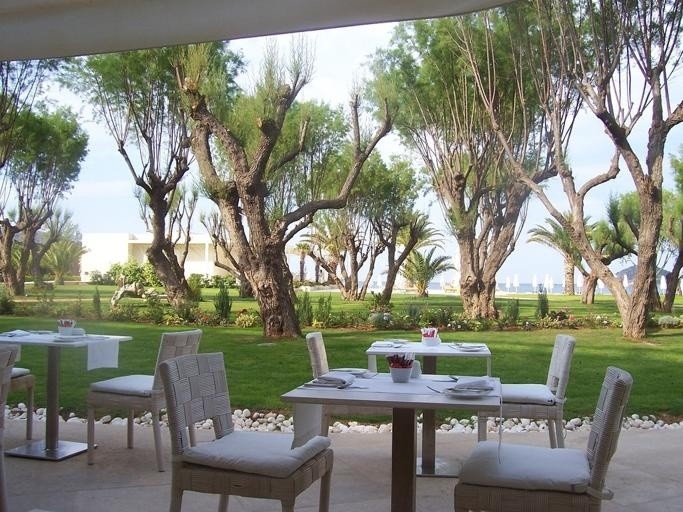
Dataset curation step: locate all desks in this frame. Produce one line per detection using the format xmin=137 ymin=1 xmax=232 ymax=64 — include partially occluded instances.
xmin=280 ymin=341 xmax=502 ymax=511
xmin=0 ymin=331 xmax=133 ymax=461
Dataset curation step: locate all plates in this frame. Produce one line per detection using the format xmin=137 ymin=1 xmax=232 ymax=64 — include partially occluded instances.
xmin=457 ymin=347 xmax=481 ymax=352
xmin=443 ymin=387 xmax=492 ymax=398
xmin=335 ymin=367 xmax=369 ymax=375
xmin=87 ymin=336 xmax=110 ymax=340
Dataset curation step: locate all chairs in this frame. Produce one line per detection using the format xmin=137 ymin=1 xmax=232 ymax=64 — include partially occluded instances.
xmin=153 ymin=350 xmax=336 ymax=512
xmin=453 ymin=332 xmax=634 ymax=511
xmin=83 ymin=330 xmax=203 ymax=473
